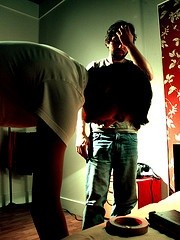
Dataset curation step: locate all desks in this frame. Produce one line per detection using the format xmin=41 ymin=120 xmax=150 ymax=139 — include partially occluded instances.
xmin=62 ymin=190 xmax=180 ymax=240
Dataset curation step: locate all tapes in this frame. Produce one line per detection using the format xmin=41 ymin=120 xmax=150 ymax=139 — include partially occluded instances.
xmin=107 ymin=215 xmax=148 ymax=235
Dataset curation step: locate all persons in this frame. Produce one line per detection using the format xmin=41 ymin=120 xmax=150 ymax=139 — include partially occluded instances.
xmin=75 ymin=19 xmax=153 ymax=231
xmin=0 ymin=42 xmax=152 ymax=240
